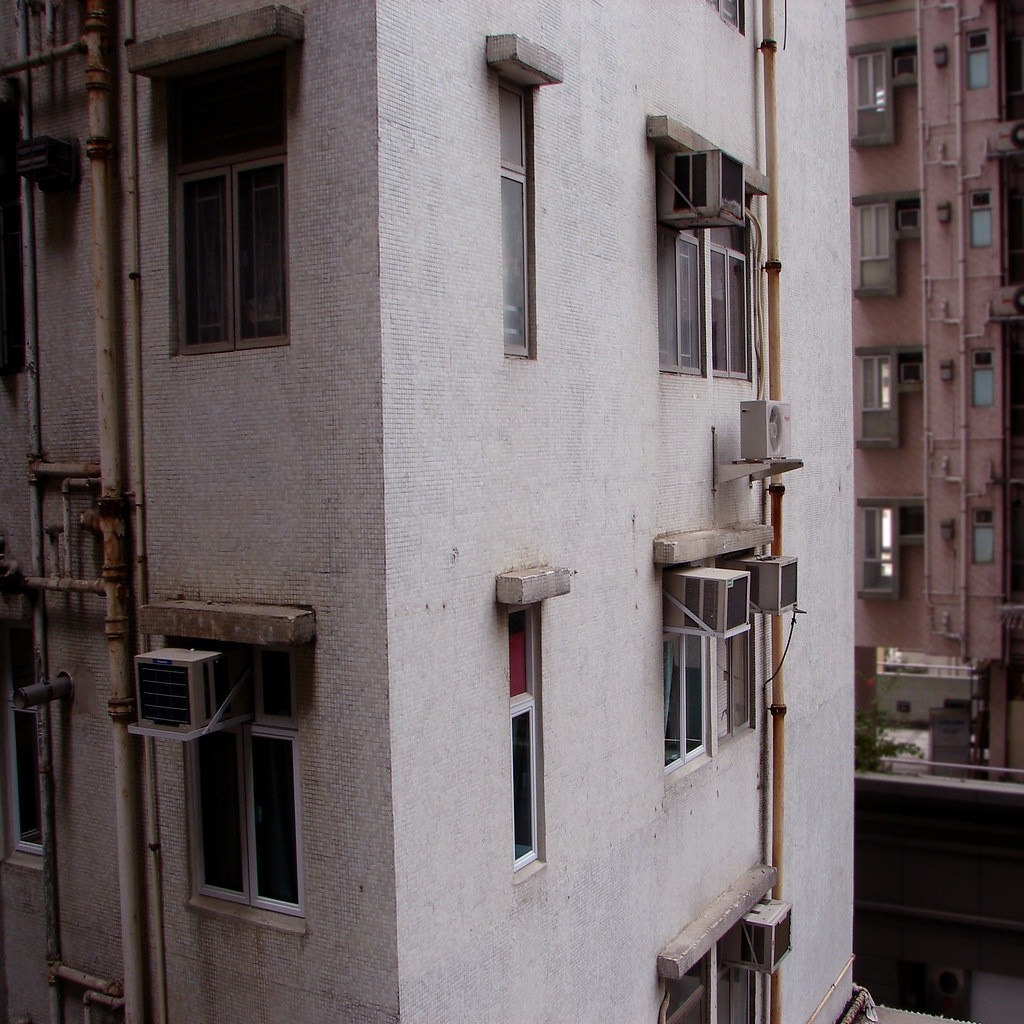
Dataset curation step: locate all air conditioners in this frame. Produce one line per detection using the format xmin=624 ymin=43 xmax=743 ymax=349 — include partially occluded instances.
xmin=133 ymin=648 xmax=250 ymax=734
xmin=739 ymin=399 xmax=792 ymax=460
xmin=991 ymin=284 xmax=1024 ymax=317
xmin=995 ymin=119 xmax=1024 ymax=152
xmin=720 ymin=898 xmax=794 ymax=970
xmin=657 ymin=148 xmax=746 ymax=228
xmin=665 ymin=565 xmax=750 ymax=633
xmin=734 ymin=554 xmax=799 ymax=616
